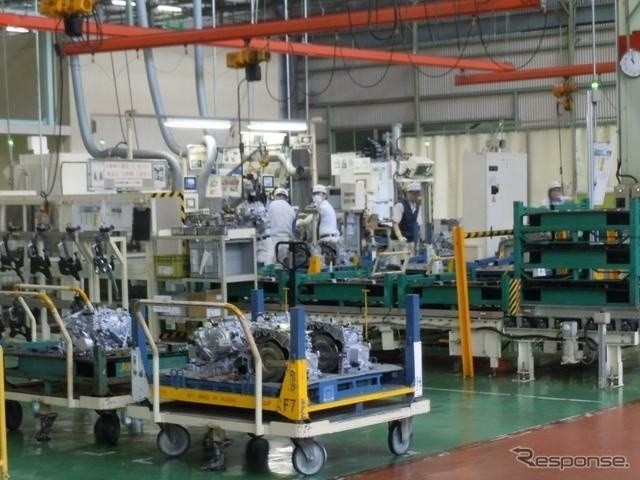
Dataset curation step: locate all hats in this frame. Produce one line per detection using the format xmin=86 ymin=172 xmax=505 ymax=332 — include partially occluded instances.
xmin=407 ymin=183 xmax=421 ymax=193
xmin=274 ymin=188 xmax=288 ymax=197
xmin=312 ymin=185 xmax=326 ymax=194
xmin=550 ymin=181 xmax=561 ymax=190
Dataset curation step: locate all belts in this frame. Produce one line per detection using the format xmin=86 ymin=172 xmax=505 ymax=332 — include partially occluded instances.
xmin=257 ymin=235 xmax=270 ymax=241
xmin=320 ymin=234 xmax=334 ymax=238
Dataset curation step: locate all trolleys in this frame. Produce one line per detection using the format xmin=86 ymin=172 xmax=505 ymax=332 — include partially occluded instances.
xmin=12 ymin=281 xmax=96 ymax=344
xmin=0 ymin=284 xmax=147 ymax=446
xmin=127 ymin=298 xmax=432 ymax=476
xmin=274 ymin=239 xmax=311 ymax=311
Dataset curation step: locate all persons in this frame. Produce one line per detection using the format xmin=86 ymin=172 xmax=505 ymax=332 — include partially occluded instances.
xmin=295 ymin=185 xmax=341 ymax=244
xmin=389 ymin=183 xmax=424 ymax=251
xmin=540 ymin=181 xmax=565 ymax=239
xmin=264 ymin=187 xmax=296 ymax=258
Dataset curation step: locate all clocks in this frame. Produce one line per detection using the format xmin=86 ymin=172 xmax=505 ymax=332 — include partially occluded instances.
xmin=620 ymin=49 xmax=640 ymax=78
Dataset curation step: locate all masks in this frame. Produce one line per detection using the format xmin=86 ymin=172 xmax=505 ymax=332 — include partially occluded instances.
xmin=312 ymin=195 xmax=324 ymax=203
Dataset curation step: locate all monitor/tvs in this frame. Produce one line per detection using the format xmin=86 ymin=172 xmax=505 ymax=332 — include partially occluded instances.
xmin=132 ymin=206 xmax=150 ymax=240
xmin=405 ymin=155 xmax=435 ymax=179
